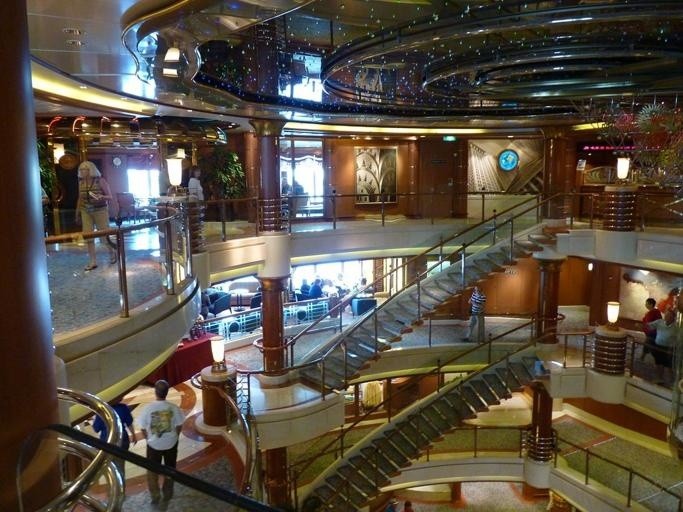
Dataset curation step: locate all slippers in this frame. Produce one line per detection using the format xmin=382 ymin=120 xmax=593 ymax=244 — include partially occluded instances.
xmin=110 ymin=247 xmax=118 ymax=264
xmin=84 ymin=263 xmax=98 ymax=270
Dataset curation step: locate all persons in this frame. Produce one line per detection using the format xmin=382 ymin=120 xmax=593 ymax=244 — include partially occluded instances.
xmin=136 ymin=380 xmax=184 ymax=503
xmin=633 ymin=310 xmax=683 ymax=383
xmin=75 ymin=160 xmax=118 ymax=270
xmin=636 ymin=297 xmax=662 ymax=361
xmin=300 ymin=275 xmax=366 ymax=298
xmin=202 ymin=292 xmax=211 ymax=319
xmin=293 ymin=181 xmax=304 ymax=195
xmin=188 ymin=165 xmax=205 ymax=220
xmin=281 ymin=177 xmax=291 ymax=194
xmin=461 ymin=285 xmax=487 ymax=342
xmin=91 ymin=403 xmax=137 ymax=494
xmin=386 ymin=498 xmax=399 ymax=512
xmin=400 ymin=500 xmax=414 ymax=512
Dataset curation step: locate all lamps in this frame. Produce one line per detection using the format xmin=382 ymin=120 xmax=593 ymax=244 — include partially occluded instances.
xmin=165 ymin=158 xmax=187 ymax=197
xmin=615 ymin=157 xmax=631 ymax=185
xmin=605 ymin=301 xmax=622 ymax=331
xmin=209 ymin=335 xmax=227 ymax=373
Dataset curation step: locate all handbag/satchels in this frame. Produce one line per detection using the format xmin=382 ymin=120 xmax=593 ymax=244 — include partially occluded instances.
xmin=93 ymin=199 xmax=108 ymax=208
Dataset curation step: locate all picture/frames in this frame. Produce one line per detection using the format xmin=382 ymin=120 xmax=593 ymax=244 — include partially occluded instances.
xmin=353 ymin=145 xmax=399 ymax=205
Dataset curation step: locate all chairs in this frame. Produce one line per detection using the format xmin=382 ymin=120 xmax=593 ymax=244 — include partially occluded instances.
xmin=115 ymin=192 xmax=159 ymax=224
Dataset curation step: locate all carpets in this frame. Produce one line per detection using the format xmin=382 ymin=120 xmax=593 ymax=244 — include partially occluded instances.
xmin=551 ymin=414 xmax=617 ymax=458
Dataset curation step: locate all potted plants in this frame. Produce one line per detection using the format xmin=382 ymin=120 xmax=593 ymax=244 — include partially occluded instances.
xmin=198 ymin=146 xmax=247 ymax=222
